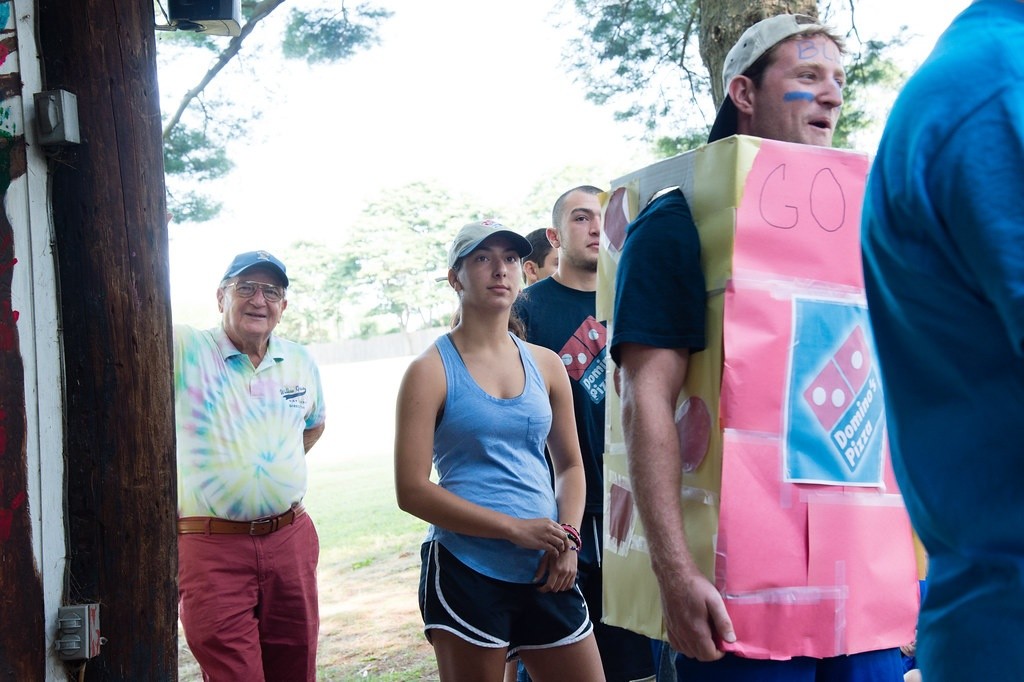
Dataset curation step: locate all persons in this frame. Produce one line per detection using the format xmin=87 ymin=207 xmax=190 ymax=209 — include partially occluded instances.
xmin=172 ymin=251 xmax=327 ymax=682
xmin=861 ymin=0 xmax=1024 ymax=682
xmin=519 ymin=226 xmax=562 ymax=286
xmin=394 ymin=222 xmax=606 ymax=682
xmin=609 ymin=13 xmax=930 ymax=682
xmin=509 ymin=184 xmax=659 ymax=682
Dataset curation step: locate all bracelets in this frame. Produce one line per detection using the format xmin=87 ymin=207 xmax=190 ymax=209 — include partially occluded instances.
xmin=560 ymin=522 xmax=582 ymax=551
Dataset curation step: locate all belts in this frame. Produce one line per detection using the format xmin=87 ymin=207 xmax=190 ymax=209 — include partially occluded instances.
xmin=177 ymin=502 xmax=307 ymax=537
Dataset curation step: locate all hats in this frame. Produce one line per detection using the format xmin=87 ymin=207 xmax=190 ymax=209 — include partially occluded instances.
xmin=220 ymin=250 xmax=289 ymax=298
xmin=448 ymin=219 xmax=534 ymax=272
xmin=706 ymin=14 xmax=826 ymax=144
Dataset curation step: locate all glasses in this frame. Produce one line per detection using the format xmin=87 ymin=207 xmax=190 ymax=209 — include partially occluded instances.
xmin=224 ymin=280 xmax=285 ymax=303
